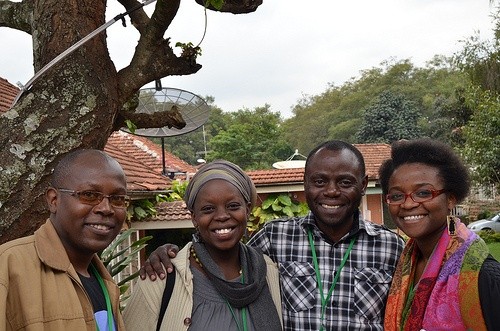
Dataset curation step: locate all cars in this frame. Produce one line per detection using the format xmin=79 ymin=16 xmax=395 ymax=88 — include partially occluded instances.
xmin=467 ymin=213 xmax=500 ymax=233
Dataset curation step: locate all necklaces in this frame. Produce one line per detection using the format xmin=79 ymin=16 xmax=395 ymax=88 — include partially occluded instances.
xmin=190 ymin=246 xmax=243 ymax=276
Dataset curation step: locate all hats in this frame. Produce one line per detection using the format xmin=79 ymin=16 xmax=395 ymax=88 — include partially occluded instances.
xmin=184 ymin=159 xmax=257 ymax=214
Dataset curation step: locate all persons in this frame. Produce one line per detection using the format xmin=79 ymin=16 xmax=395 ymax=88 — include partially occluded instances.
xmin=0 ymin=148 xmax=129 ymax=331
xmin=122 ymin=159 xmax=284 ymax=331
xmin=379 ymin=139 xmax=500 ymax=331
xmin=140 ymin=140 xmax=406 ymax=331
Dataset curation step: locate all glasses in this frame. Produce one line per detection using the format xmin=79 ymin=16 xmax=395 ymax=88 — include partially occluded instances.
xmin=58 ymin=189 xmax=131 ymax=208
xmin=383 ymin=189 xmax=445 ymax=205
